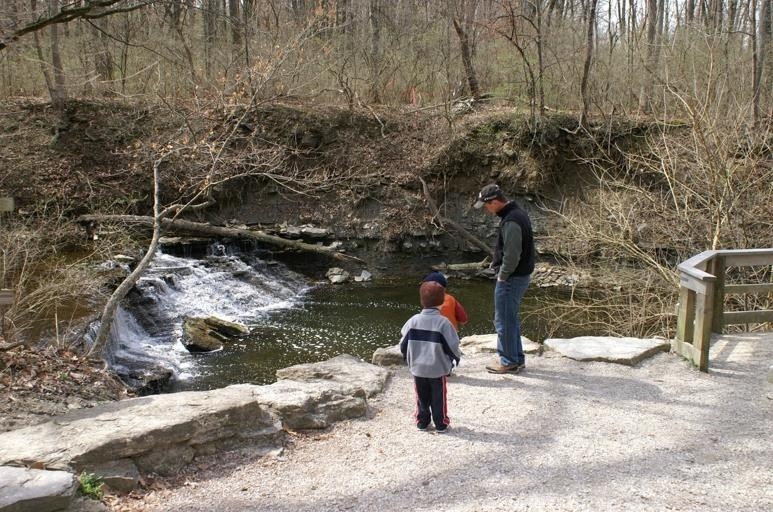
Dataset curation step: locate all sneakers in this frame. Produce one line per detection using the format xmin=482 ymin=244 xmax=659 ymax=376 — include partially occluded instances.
xmin=418 ymin=420 xmax=450 ymax=432
xmin=486 ymin=356 xmax=526 ymax=374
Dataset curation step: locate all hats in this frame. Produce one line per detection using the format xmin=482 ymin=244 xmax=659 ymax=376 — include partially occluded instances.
xmin=473 ymin=183 xmax=504 ymax=210
xmin=418 ymin=271 xmax=448 ymax=307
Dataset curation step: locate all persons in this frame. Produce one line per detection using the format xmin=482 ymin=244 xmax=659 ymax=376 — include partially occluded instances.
xmin=398 ymin=280 xmax=463 ymax=432
xmin=424 ymin=271 xmax=468 ymax=378
xmin=472 ymin=182 xmax=534 ymax=374
